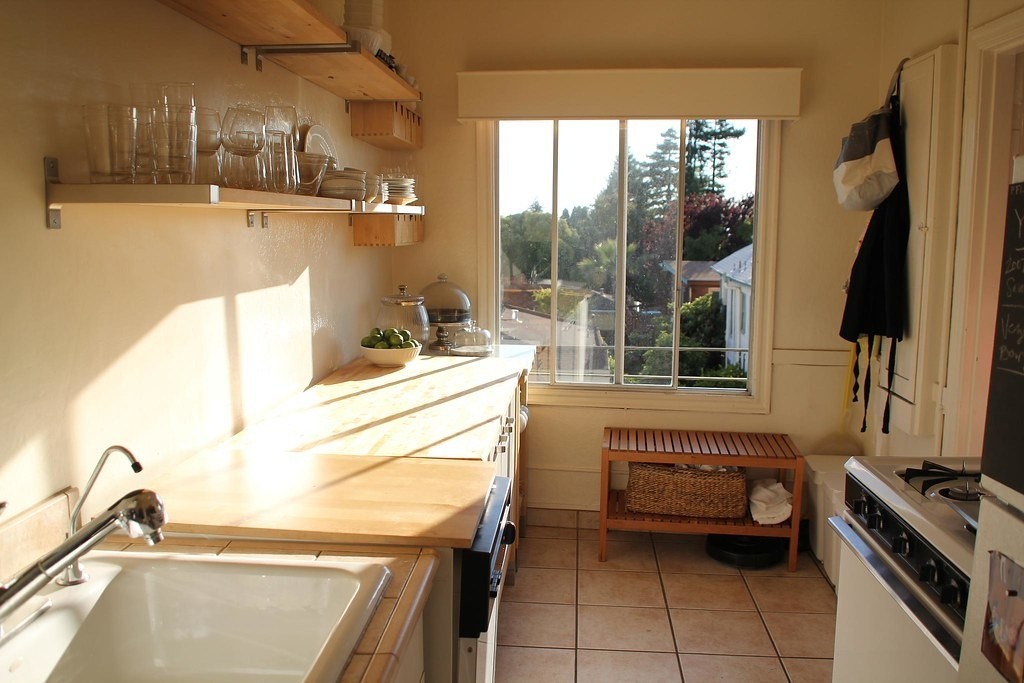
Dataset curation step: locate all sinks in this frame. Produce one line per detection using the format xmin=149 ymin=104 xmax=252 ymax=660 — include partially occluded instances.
xmin=2 ymin=550 xmax=389 ymax=683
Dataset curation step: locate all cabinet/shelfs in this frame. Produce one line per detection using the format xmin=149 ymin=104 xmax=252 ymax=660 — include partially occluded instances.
xmin=599 ymin=427 xmax=806 ymax=573
xmin=485 ymin=375 xmax=529 ymax=586
xmin=44 ymin=0 xmax=426 ymax=247
xmin=874 ymin=45 xmax=956 ymax=438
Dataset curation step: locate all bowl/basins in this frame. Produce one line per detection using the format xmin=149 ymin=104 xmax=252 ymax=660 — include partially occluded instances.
xmin=360 ymin=343 xmax=422 ymax=368
xmin=341 ymin=0 xmax=387 ymax=56
xmin=343 ymin=167 xmax=380 ymax=204
xmin=381 ymin=31 xmax=392 ymax=54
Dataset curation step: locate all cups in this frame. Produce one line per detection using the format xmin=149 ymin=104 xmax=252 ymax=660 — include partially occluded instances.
xmin=76 ymin=80 xmax=331 ymax=196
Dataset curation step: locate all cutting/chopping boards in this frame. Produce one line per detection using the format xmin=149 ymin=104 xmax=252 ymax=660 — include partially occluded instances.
xmin=144 ymin=449 xmax=499 ymax=548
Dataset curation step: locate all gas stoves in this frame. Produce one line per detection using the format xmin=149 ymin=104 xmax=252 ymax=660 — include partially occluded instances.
xmin=840 ymin=454 xmax=987 ymax=625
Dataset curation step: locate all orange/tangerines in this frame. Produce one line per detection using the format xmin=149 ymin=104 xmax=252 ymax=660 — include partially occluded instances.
xmin=361 ymin=328 xmax=419 ymax=349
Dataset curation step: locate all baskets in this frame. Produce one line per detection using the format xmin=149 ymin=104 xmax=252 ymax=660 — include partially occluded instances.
xmin=622 ymin=462 xmax=747 ymax=521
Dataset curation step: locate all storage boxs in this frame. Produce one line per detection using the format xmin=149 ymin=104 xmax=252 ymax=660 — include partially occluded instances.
xmin=804 ymin=455 xmax=850 ymax=598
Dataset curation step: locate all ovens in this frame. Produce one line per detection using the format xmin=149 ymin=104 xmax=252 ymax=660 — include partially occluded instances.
xmin=454 ymin=474 xmax=517 ymax=683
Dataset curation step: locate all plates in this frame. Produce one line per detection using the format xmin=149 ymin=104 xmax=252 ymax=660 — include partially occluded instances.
xmin=320 ymin=170 xmax=367 ymax=200
xmin=303 ymin=124 xmax=339 ymax=169
xmin=383 ymin=177 xmax=419 ymax=205
xmin=379 ymin=174 xmax=389 ymax=203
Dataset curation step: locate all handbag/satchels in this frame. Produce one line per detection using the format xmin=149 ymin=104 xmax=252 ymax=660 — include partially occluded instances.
xmin=831 ymin=56 xmax=908 ymax=211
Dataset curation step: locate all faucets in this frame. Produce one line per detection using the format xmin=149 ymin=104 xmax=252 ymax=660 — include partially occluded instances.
xmin=62 ymin=443 xmax=143 ymax=583
xmin=0 ymin=489 xmax=170 ymax=619
